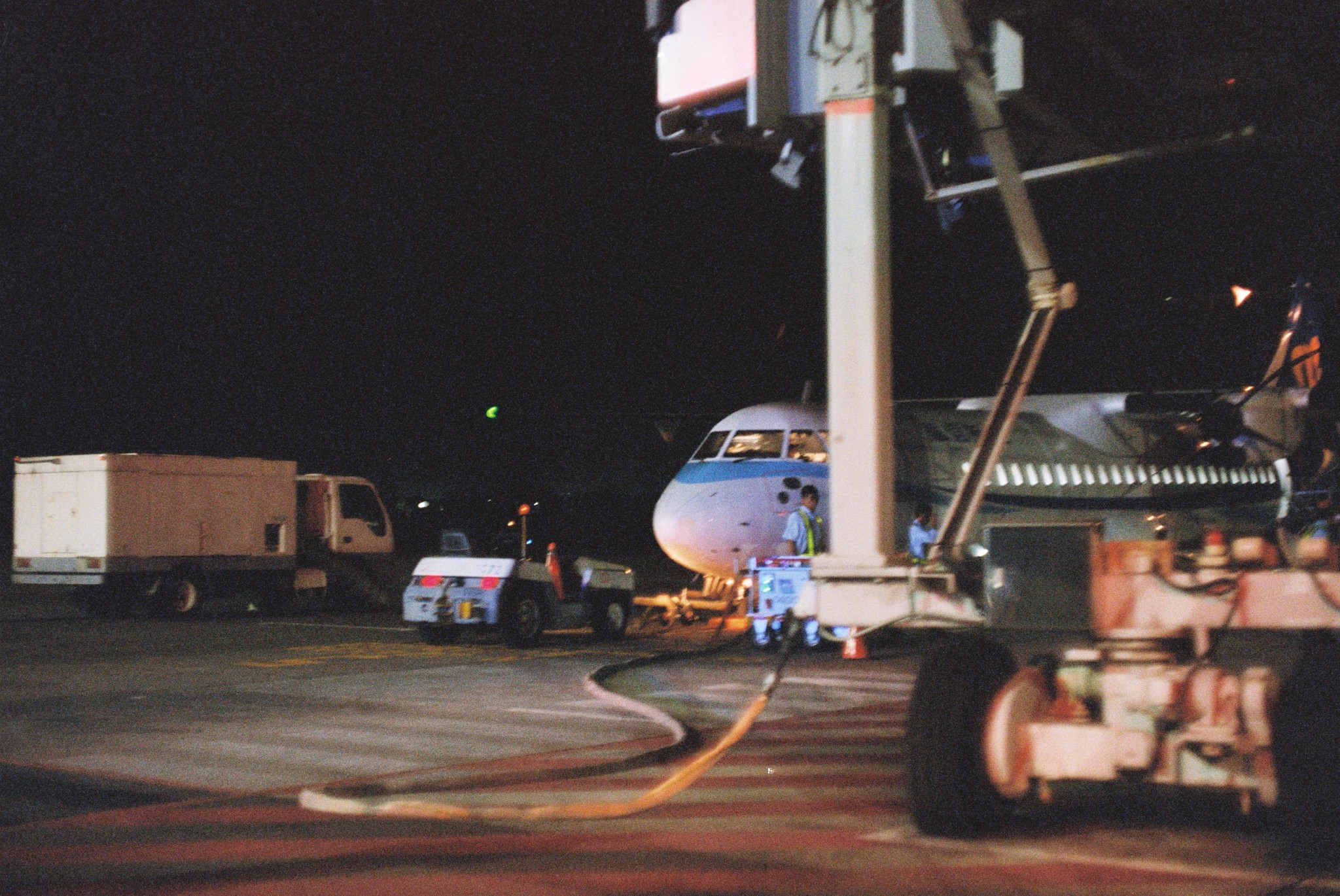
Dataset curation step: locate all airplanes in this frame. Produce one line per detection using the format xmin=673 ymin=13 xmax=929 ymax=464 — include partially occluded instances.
xmin=651 ymin=276 xmax=1340 ymax=639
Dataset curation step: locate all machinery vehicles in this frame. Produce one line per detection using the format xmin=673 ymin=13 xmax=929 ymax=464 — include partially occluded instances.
xmin=401 ymin=502 xmax=635 ymax=650
xmin=896 ymin=277 xmax=1340 ymax=861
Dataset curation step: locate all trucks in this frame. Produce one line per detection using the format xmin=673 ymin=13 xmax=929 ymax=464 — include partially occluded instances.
xmin=12 ymin=453 xmax=398 ymax=621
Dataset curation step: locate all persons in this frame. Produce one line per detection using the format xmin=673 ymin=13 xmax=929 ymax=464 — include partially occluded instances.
xmin=781 ymin=484 xmax=850 ymax=649
xmin=752 ymin=614 xmax=781 ymax=647
xmin=909 ymin=504 xmax=939 ymax=565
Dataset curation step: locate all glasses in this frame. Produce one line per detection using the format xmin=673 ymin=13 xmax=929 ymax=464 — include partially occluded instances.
xmin=804 ymin=496 xmax=819 ymax=501
